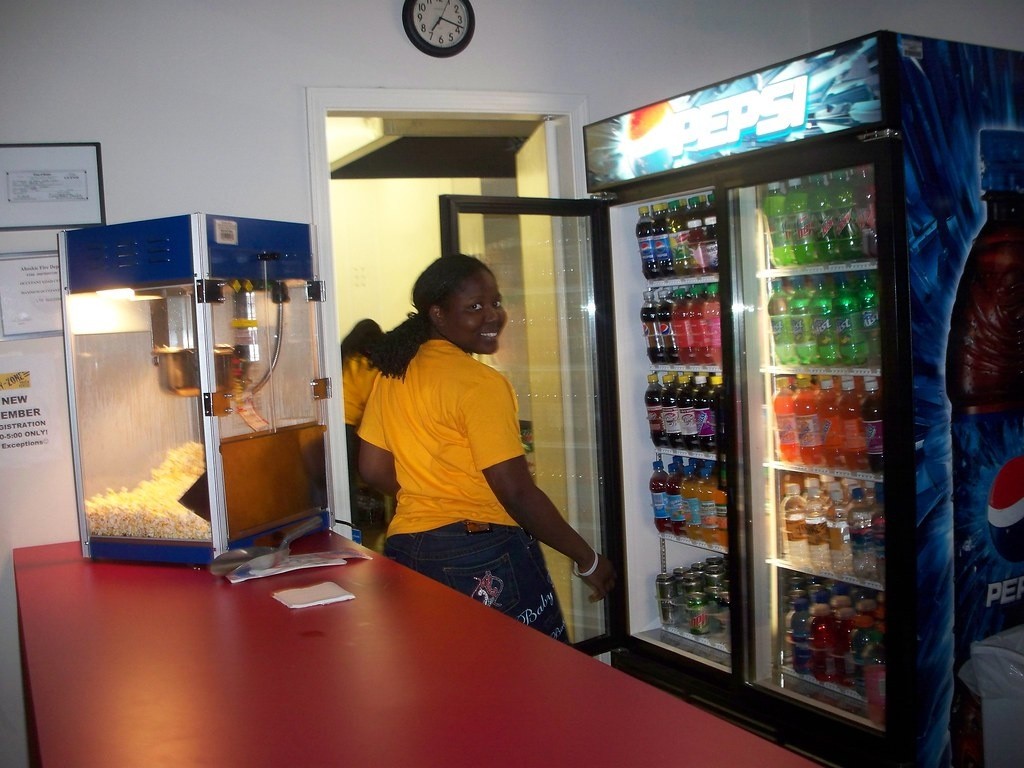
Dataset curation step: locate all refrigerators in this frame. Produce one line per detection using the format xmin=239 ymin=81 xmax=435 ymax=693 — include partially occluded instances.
xmin=438 ymin=30 xmax=1023 ymax=768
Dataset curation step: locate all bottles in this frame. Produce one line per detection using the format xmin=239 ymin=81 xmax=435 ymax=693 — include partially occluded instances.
xmin=636 ymin=164 xmax=887 ymax=726
xmin=945 ymin=128 xmax=1024 ymax=768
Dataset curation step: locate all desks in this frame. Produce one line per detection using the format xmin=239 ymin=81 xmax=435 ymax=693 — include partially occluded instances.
xmin=12 ymin=540 xmax=823 ymax=768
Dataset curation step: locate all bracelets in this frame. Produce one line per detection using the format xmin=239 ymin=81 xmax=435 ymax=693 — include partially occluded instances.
xmin=574 ymin=547 xmax=599 ymax=578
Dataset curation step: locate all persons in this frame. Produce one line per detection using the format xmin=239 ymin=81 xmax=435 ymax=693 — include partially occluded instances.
xmin=356 ymin=253 xmax=618 ymax=645
xmin=341 ymin=319 xmax=382 ymax=470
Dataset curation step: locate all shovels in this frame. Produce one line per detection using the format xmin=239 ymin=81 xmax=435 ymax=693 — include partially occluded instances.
xmin=208 ymin=515 xmax=324 ymax=578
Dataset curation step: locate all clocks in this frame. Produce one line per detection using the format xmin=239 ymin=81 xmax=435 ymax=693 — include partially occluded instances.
xmin=402 ymin=0 xmax=475 ymax=58
